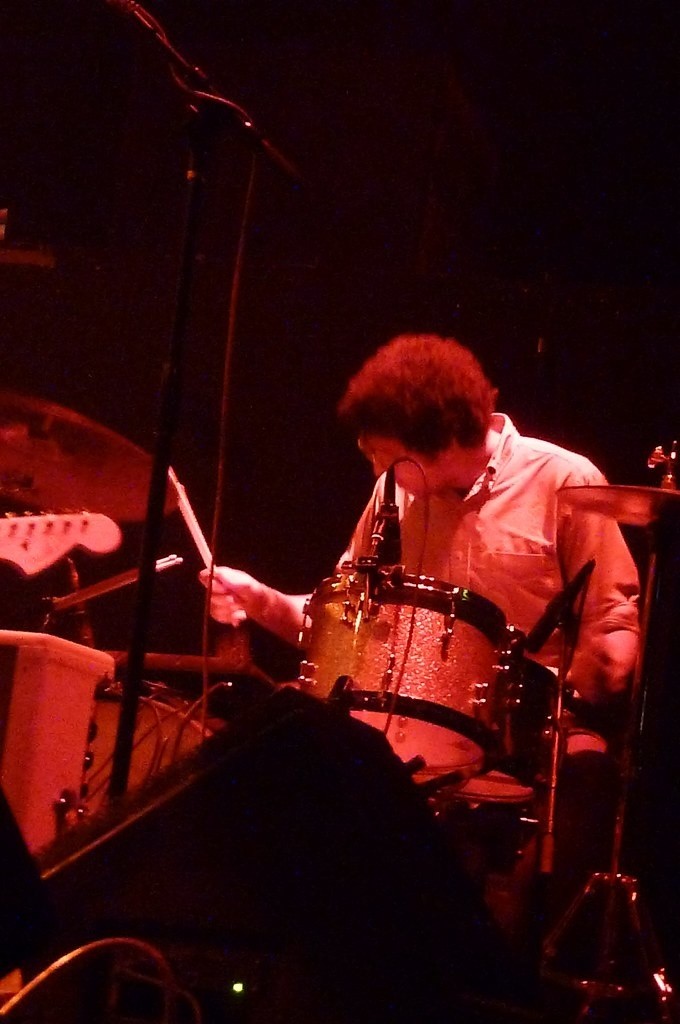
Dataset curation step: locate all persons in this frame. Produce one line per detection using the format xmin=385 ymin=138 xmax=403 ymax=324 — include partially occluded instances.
xmin=198 ymin=332 xmax=642 ymax=932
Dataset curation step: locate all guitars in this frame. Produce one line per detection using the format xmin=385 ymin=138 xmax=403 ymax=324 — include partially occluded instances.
xmin=0 ymin=505 xmax=133 ymax=590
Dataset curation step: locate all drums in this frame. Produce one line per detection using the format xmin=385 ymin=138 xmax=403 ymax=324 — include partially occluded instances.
xmin=283 ymin=565 xmax=516 ymax=791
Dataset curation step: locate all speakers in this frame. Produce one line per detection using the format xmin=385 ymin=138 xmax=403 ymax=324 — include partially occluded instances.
xmin=24 ymin=687 xmax=519 ymax=1024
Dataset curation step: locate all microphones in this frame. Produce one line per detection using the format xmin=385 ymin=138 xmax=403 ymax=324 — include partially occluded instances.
xmin=524 ymin=558 xmax=595 ymax=654
xmin=377 ymin=466 xmax=403 ymax=567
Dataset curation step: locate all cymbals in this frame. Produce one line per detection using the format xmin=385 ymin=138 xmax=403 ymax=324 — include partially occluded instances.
xmin=0 ymin=383 xmax=196 ymax=529
xmin=552 ymin=477 xmax=680 ymax=532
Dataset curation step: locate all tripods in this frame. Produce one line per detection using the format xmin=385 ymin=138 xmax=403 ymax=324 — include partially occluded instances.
xmin=532 ymin=553 xmax=680 ymax=1024
